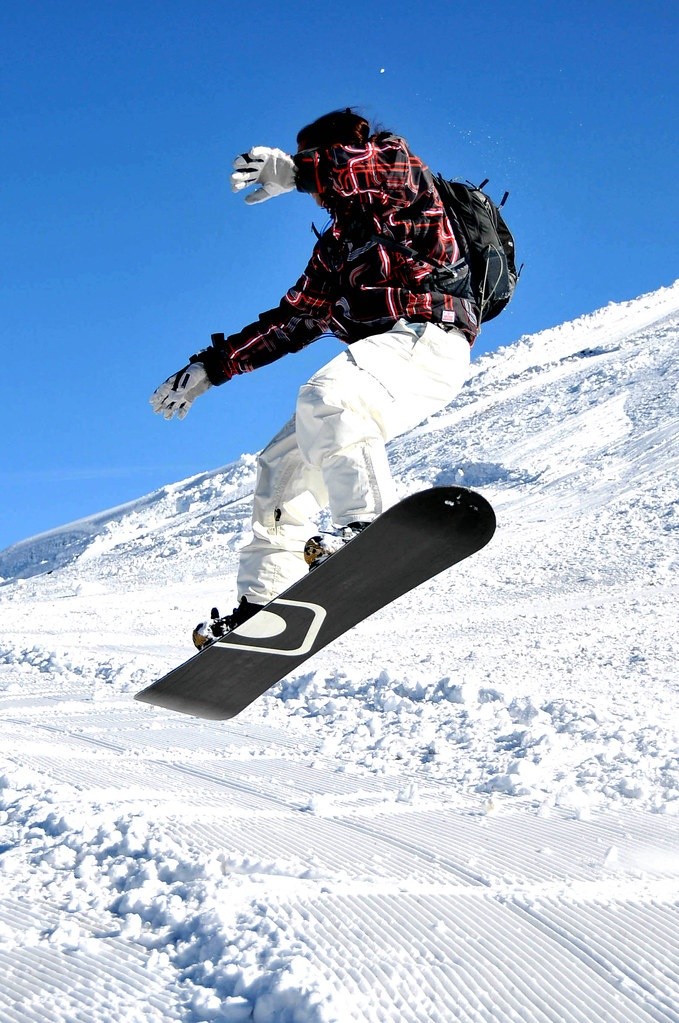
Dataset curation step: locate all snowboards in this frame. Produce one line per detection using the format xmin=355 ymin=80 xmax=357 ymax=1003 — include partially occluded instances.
xmin=126 ymin=482 xmax=498 ymax=720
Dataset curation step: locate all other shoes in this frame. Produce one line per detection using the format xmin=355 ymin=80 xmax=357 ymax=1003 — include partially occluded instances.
xmin=304 ymin=523 xmax=368 ymax=566
xmin=192 ymin=595 xmax=260 ymax=650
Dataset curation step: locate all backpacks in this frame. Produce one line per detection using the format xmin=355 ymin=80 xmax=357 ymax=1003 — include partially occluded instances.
xmin=356 ymin=168 xmax=519 ymax=323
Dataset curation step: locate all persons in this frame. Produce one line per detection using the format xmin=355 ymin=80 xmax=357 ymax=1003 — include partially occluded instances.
xmin=150 ymin=109 xmax=476 ymax=653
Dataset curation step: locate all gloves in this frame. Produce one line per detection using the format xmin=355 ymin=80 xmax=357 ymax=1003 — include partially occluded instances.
xmin=228 ymin=145 xmax=302 ymax=205
xmin=148 ymin=362 xmax=214 ymax=421
